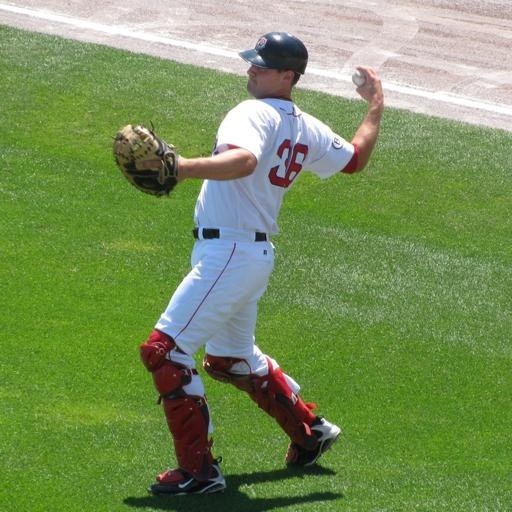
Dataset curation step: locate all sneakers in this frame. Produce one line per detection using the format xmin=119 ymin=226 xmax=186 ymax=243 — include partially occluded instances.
xmin=285 ymin=416 xmax=341 ymax=467
xmin=146 ymin=456 xmax=227 ymax=496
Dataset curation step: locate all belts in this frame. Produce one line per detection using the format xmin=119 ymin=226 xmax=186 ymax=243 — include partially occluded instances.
xmin=192 ymin=227 xmax=266 ymax=241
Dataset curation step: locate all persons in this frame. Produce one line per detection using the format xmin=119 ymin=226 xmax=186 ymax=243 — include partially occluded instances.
xmin=139 ymin=32 xmax=386 ymax=496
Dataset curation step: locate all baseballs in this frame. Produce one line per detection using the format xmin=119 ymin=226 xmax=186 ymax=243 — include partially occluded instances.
xmin=352 ymin=71 xmax=368 ymax=86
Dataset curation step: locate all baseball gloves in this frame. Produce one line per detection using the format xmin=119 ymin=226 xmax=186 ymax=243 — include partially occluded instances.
xmin=113 ymin=124 xmax=178 ymax=197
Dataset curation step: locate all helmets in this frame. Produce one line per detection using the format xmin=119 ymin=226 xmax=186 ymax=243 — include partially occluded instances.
xmin=238 ymin=32 xmax=308 ymax=74
